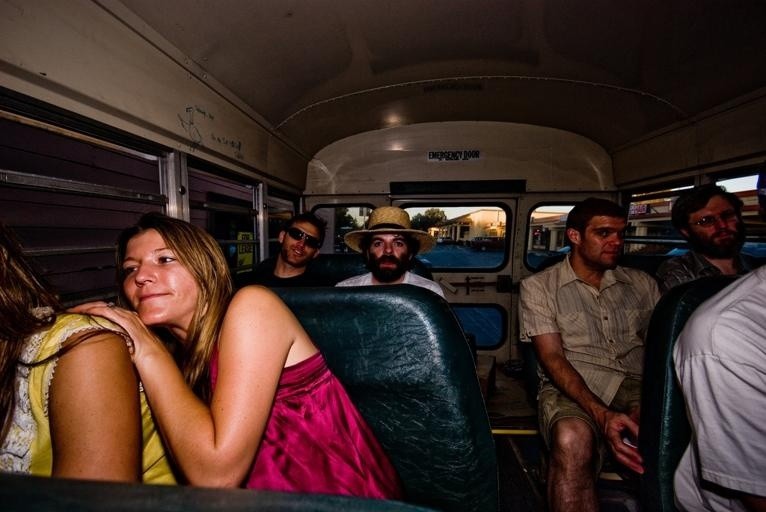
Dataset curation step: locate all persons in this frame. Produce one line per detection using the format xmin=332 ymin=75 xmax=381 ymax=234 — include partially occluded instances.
xmin=64 ymin=211 xmax=407 ymax=500
xmin=235 ymin=210 xmax=345 ymax=288
xmin=672 ymin=170 xmax=766 ymax=512
xmin=0 ymin=229 xmax=183 ymax=487
xmin=515 ymin=197 xmax=661 ymax=512
xmin=334 ymin=208 xmax=447 ymax=300
xmin=648 ymin=185 xmax=762 ymax=293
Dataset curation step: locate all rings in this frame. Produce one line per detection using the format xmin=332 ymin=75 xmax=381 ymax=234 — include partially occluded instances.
xmin=105 ymin=301 xmax=115 ymax=309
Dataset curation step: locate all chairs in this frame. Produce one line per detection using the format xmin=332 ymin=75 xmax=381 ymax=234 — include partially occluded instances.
xmin=2 ymin=262 xmax=500 ymax=509
xmin=522 ymin=249 xmax=765 ymax=511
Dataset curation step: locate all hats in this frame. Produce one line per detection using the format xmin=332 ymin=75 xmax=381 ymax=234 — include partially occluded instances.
xmin=341 ymin=205 xmax=436 ymax=258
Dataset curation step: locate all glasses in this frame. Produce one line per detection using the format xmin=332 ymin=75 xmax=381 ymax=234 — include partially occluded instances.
xmin=284 ymin=226 xmax=322 ymax=250
xmin=687 ymin=209 xmax=737 ymax=229
